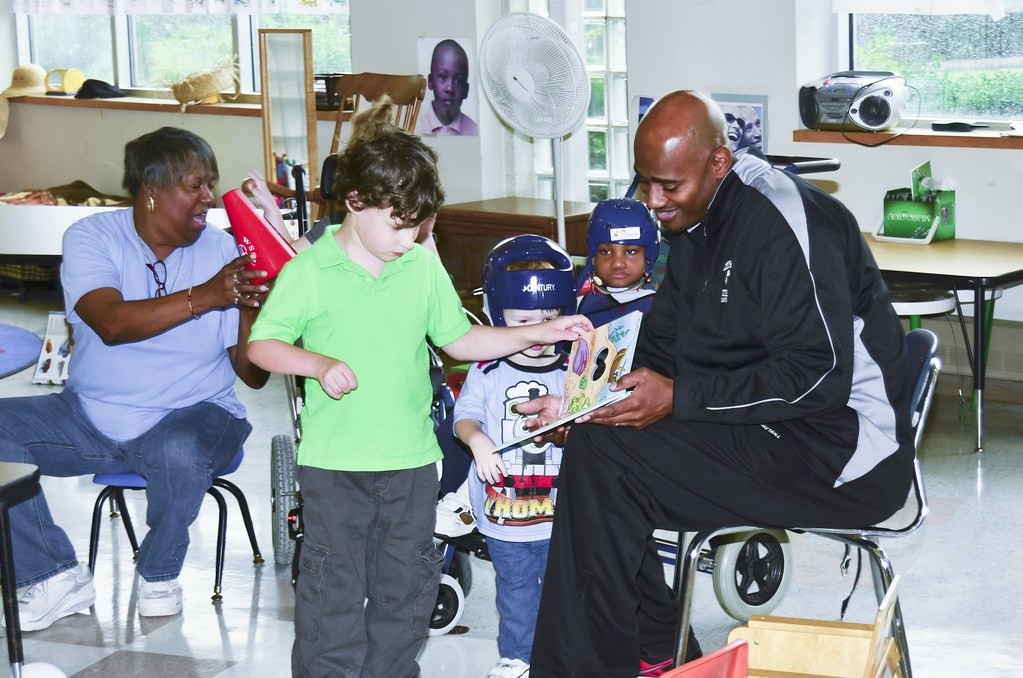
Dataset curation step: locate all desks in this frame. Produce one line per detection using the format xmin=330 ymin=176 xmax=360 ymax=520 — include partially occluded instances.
xmin=860 ymin=231 xmax=1023 ymax=453
xmin=0 ymin=202 xmax=293 ymax=257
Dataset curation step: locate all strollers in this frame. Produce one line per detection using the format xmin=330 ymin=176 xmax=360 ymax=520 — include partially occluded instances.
xmin=268 ymin=148 xmax=469 ymax=566
xmin=426 ymin=152 xmax=841 ymax=638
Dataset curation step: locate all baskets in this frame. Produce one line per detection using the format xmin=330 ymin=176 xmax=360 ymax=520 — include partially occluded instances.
xmin=155 ymin=53 xmax=243 ymax=113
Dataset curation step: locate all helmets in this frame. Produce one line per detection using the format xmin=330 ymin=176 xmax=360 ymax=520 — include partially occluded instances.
xmin=585 ymin=197 xmax=661 ymax=296
xmin=472 ymin=233 xmax=579 ymax=356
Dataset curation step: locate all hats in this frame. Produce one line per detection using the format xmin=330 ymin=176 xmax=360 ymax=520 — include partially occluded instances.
xmin=1 ymin=64 xmax=58 ymax=98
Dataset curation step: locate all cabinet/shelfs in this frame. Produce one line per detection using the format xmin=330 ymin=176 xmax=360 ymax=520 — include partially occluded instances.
xmin=431 ymin=194 xmax=593 ymax=325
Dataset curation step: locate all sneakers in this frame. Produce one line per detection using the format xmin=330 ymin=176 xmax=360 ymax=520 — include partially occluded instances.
xmin=1 ymin=561 xmax=97 ymax=632
xmin=138 ymin=572 xmax=184 ymax=617
xmin=487 ymin=657 xmax=530 ymax=678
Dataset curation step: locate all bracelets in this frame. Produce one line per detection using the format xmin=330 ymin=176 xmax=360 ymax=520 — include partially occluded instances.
xmin=188 ymin=288 xmax=201 ymax=319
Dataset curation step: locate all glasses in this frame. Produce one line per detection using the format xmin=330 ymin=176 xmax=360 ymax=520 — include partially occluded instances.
xmin=147 ymin=259 xmax=168 ymax=298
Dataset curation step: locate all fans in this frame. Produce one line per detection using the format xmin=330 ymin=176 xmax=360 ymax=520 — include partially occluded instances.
xmin=479 ymin=10 xmax=591 ymax=252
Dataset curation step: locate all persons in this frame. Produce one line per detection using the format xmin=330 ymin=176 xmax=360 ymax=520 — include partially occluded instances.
xmin=246 ymin=125 xmax=595 ymax=678
xmin=721 ymin=105 xmax=763 ymax=151
xmin=530 ymin=90 xmax=915 ymax=678
xmin=450 ymin=233 xmax=578 ymax=678
xmin=0 ymin=127 xmax=278 ymax=631
xmin=577 ymin=198 xmax=659 ymax=326
xmin=418 ymin=39 xmax=478 ymax=136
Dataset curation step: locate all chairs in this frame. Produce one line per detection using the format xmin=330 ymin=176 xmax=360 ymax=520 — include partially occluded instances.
xmin=673 ymin=325 xmax=942 ymax=678
xmin=318 ymin=73 xmax=427 ymax=224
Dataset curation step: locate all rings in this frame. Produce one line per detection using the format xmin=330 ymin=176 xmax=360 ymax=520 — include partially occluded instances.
xmin=233 ymin=273 xmax=239 ymax=283
xmin=234 ymin=298 xmax=239 ymax=305
xmin=233 ymin=286 xmax=241 ymax=296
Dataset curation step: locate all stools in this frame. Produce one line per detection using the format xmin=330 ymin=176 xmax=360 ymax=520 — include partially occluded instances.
xmin=951 ymin=286 xmax=1005 ymax=412
xmin=86 ymin=448 xmax=265 ymax=602
xmin=883 ymin=281 xmax=955 ymax=331
xmin=0 ymin=462 xmax=41 ymax=678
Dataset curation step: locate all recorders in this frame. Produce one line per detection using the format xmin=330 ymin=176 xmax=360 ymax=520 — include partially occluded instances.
xmin=799 ymin=71 xmax=907 ymax=133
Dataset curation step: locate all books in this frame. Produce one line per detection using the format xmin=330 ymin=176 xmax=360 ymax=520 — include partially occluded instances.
xmin=494 ymin=309 xmax=642 ymax=452
